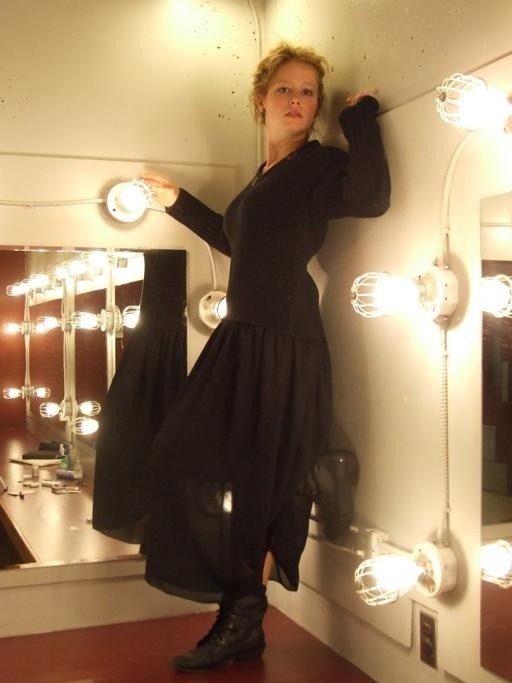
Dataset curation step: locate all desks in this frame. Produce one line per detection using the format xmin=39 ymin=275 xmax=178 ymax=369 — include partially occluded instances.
xmin=0 ymin=429 xmax=143 ymax=562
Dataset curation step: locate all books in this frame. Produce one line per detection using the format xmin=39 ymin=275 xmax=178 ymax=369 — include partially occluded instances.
xmin=8 ymin=455 xmax=63 ymax=467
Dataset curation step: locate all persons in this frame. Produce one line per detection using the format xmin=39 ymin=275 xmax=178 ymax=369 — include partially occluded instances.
xmin=132 ymin=40 xmax=392 ymax=674
xmin=89 ymin=248 xmax=187 ymax=543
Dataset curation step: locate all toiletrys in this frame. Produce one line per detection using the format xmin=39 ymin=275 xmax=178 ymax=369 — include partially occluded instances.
xmin=30 ymin=442 xmax=85 ymax=481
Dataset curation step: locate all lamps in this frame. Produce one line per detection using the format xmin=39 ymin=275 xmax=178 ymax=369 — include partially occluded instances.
xmin=343 ymin=265 xmax=462 ymax=324
xmin=481 ymin=274 xmax=512 ymax=321
xmin=434 ymin=69 xmax=512 ymax=139
xmin=109 ymin=179 xmax=150 ymax=223
xmin=480 ymin=539 xmax=511 ymax=590
xmin=2 ymin=255 xmax=146 ymax=436
xmin=352 ymin=541 xmax=458 ymax=607
xmin=196 ymin=290 xmax=232 ymax=329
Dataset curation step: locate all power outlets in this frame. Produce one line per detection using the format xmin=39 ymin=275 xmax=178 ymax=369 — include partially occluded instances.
xmin=419 ymin=611 xmax=437 ymax=669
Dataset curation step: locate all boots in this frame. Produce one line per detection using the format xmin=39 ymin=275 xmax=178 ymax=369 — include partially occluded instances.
xmin=298 ymin=449 xmax=361 ymax=541
xmin=170 ymin=591 xmax=269 ymax=674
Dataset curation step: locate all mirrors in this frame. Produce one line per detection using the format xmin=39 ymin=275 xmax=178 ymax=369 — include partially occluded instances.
xmin=1 ymin=249 xmax=187 ymax=574
xmin=477 ymin=189 xmax=512 ymax=683
